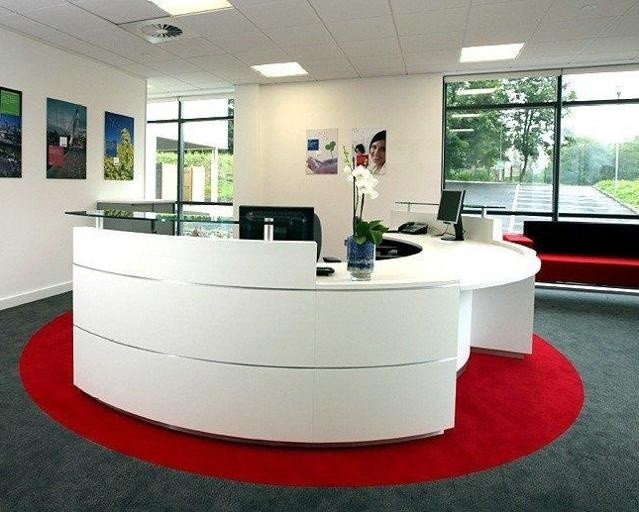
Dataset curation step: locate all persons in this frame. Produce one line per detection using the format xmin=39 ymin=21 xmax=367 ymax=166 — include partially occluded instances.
xmin=354 ymin=144 xmax=368 ymax=168
xmin=368 ymin=131 xmax=386 ymax=175
xmin=306 ymin=155 xmax=338 ymax=175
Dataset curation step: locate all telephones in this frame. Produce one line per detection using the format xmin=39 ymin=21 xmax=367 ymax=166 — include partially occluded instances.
xmin=398 ymin=222 xmax=429 ymax=234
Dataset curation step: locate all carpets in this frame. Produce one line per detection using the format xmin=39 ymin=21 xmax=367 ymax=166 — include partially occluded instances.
xmin=20 ymin=311 xmax=584 ymax=488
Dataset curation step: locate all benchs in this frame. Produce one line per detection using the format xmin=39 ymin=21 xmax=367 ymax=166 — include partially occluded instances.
xmin=502 ymin=221 xmax=639 ymax=285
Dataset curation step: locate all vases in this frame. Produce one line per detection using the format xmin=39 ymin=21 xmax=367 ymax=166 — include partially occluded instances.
xmin=346 ymin=237 xmax=376 ymax=281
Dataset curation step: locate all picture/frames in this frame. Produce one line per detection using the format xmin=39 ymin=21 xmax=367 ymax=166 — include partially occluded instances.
xmin=0 ymin=86 xmax=22 ymax=178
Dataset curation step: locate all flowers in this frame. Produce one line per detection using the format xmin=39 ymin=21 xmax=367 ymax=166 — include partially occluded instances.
xmin=342 ymin=143 xmax=388 ymax=245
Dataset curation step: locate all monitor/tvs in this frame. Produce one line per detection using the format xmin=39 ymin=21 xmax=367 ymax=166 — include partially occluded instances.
xmin=436 ymin=188 xmax=466 ymax=242
xmin=238 ymin=205 xmax=314 ymax=241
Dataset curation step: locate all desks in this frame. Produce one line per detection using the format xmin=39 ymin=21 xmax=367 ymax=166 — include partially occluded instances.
xmin=72 ymin=225 xmax=542 ymax=449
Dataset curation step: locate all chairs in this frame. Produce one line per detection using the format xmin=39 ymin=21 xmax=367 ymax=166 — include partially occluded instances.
xmin=314 ymin=214 xmax=322 ymax=262
